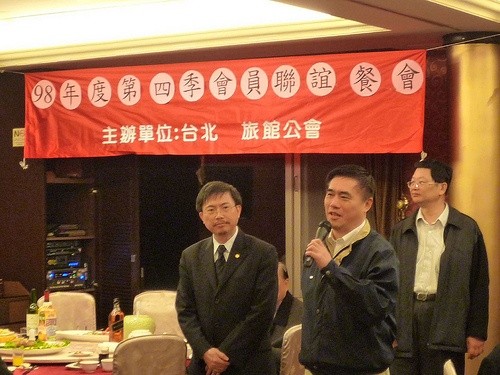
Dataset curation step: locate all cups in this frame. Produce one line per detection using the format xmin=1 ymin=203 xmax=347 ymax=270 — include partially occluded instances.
xmin=13 ymin=348 xmax=24 ymax=366
xmin=98 ymin=346 xmax=109 ymax=365
xmin=101 ymin=359 xmax=113 ymax=371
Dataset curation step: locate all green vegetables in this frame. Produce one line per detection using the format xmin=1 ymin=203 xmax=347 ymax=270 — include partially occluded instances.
xmin=2 ymin=337 xmax=71 ymax=350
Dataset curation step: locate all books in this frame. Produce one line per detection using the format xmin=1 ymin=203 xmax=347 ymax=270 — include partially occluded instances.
xmin=46 ymin=224 xmax=85 ymax=237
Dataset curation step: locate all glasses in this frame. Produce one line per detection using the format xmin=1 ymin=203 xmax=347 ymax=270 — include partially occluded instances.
xmin=408 ymin=182 xmax=440 ymax=188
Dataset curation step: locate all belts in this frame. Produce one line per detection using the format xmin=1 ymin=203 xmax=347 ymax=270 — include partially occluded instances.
xmin=413 ymin=292 xmax=436 ymax=301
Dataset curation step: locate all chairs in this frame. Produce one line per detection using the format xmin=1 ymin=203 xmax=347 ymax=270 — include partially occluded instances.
xmin=37 ymin=291 xmax=96 ymax=332
xmin=134 ymin=291 xmax=187 ymax=342
xmin=280 ymin=324 xmax=304 ymax=375
xmin=112 ymin=335 xmax=187 ymax=375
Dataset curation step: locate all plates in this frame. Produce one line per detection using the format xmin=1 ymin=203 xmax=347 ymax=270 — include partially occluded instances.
xmin=55 ymin=330 xmax=109 ymax=342
xmin=69 ymin=352 xmax=93 ymax=356
xmin=65 ymin=364 xmax=100 ymax=369
xmin=0 ymin=340 xmax=70 ymax=354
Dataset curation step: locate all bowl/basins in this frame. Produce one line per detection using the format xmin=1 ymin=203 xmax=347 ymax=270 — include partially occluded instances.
xmin=78 ymin=361 xmax=99 ymax=373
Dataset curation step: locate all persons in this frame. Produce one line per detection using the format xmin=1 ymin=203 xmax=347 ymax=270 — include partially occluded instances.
xmin=298 ymin=164 xmax=399 ymax=375
xmin=389 ymin=159 xmax=489 ymax=375
xmin=478 ymin=343 xmax=500 ymax=375
xmin=266 ymin=262 xmax=304 ymax=375
xmin=174 ymin=182 xmax=279 ymax=375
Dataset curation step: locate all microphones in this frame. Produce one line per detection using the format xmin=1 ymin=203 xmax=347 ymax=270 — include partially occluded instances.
xmin=304 ymin=220 xmax=332 ymax=267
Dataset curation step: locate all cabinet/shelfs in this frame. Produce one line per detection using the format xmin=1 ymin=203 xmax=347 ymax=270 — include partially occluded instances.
xmin=45 ymin=178 xmax=103 ymax=330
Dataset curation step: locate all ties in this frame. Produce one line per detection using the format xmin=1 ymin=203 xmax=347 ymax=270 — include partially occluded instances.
xmin=214 ymin=245 xmax=227 ymax=286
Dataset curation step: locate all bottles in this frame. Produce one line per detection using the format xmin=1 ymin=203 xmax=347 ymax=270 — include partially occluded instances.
xmin=108 ymin=298 xmax=124 ymax=343
xmin=26 ymin=288 xmax=55 ymax=342
